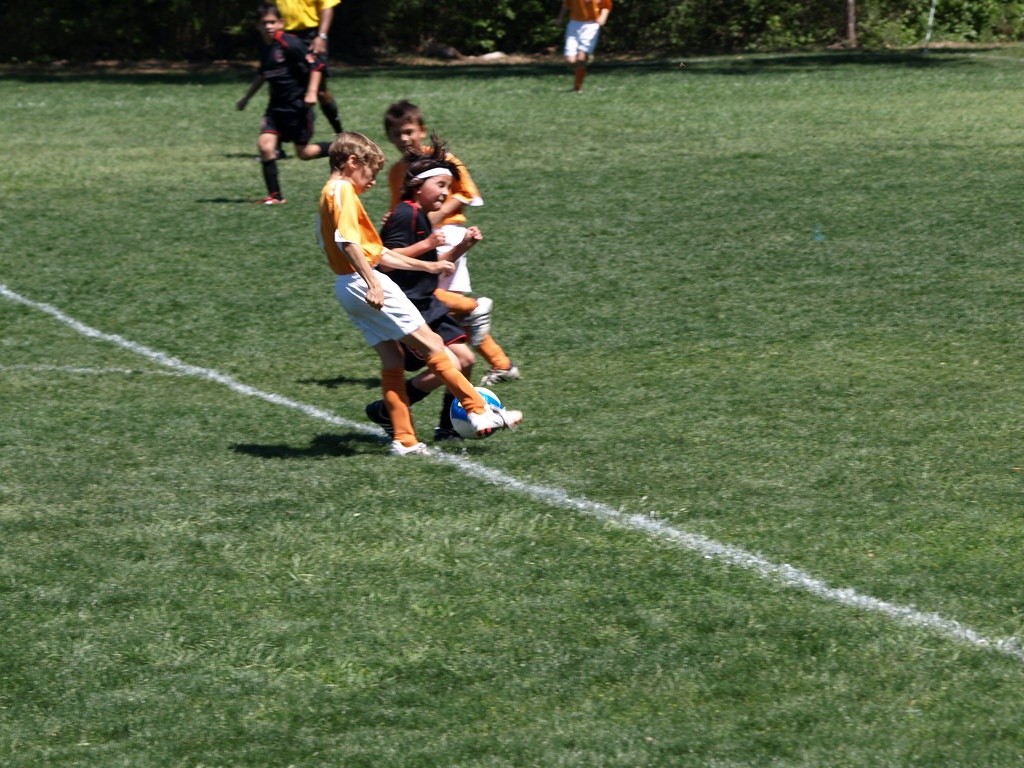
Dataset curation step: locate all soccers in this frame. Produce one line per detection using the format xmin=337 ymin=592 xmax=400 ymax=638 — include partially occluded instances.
xmin=449 ymin=386 xmax=503 ymax=440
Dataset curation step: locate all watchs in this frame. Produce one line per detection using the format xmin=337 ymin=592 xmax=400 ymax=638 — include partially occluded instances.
xmin=317 ymin=33 xmax=328 ymax=40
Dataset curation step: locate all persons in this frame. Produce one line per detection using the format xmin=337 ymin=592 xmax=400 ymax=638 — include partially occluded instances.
xmin=268 ymin=0 xmax=343 ymax=159
xmin=556 ymin=0 xmax=612 ymax=93
xmin=236 ymin=8 xmax=333 ymax=205
xmin=318 ymin=131 xmax=524 ymax=456
xmin=382 ymin=99 xmax=521 ymax=389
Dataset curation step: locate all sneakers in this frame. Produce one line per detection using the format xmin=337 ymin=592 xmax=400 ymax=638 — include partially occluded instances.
xmin=256 ymin=192 xmax=287 ymax=206
xmin=366 ymin=401 xmax=396 ymax=439
xmin=480 ymin=368 xmax=522 ymax=387
xmin=433 ymin=427 xmax=465 ymax=441
xmin=385 ymin=440 xmax=431 ymax=458
xmin=466 ymin=402 xmax=523 ymax=437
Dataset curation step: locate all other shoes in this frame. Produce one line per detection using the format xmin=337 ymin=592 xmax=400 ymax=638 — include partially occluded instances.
xmin=256 ymin=148 xmax=286 ymax=161
